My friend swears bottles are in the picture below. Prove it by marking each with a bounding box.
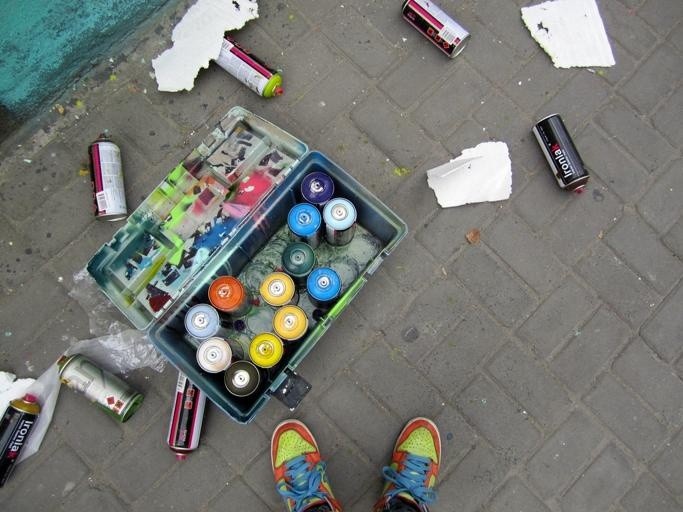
[57,354,146,424]
[89,132,129,223]
[280,242,319,289]
[306,267,343,310]
[322,196,357,247]
[299,171,335,207]
[165,371,208,461]
[286,202,322,252]
[531,112,590,196]
[0,393,41,489]
[401,0,472,59]
[182,271,309,401]
[212,34,283,100]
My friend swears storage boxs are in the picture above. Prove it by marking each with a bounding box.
[82,105,415,428]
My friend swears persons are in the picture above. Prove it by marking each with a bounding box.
[269,415,443,512]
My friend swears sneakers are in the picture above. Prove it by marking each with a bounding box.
[271,418,342,512]
[377,416,441,512]
